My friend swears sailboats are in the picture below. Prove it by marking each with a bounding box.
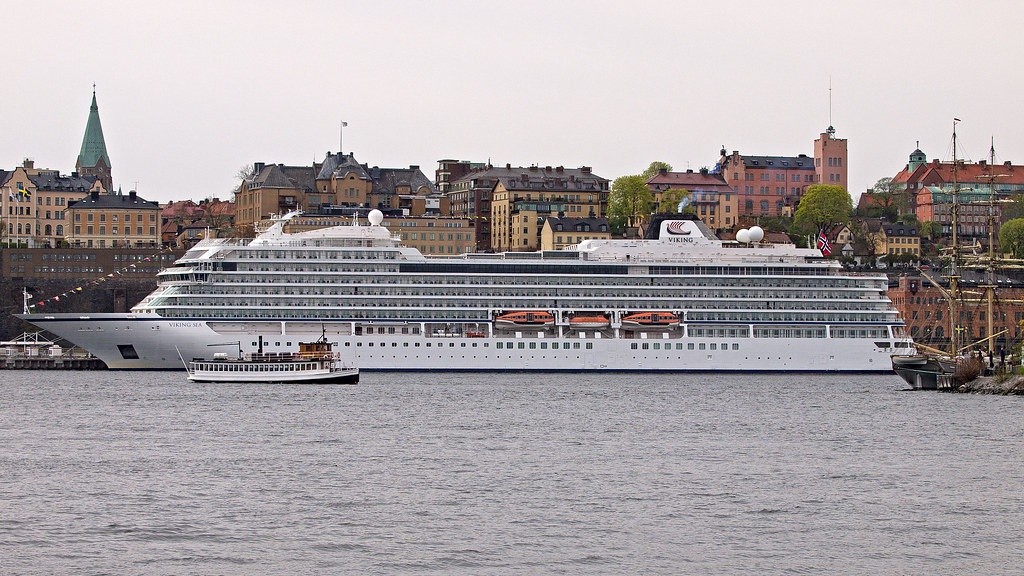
[971,134,1008,375]
[893,120,973,391]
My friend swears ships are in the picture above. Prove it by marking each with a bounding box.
[14,209,923,376]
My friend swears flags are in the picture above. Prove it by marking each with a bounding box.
[817,232,833,256]
[342,122,347,127]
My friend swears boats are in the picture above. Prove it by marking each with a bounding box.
[622,311,679,323]
[493,310,554,330]
[569,313,609,328]
[185,322,361,387]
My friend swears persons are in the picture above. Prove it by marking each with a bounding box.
[1000,348,1005,365]
[978,349,982,357]
[987,349,994,368]
